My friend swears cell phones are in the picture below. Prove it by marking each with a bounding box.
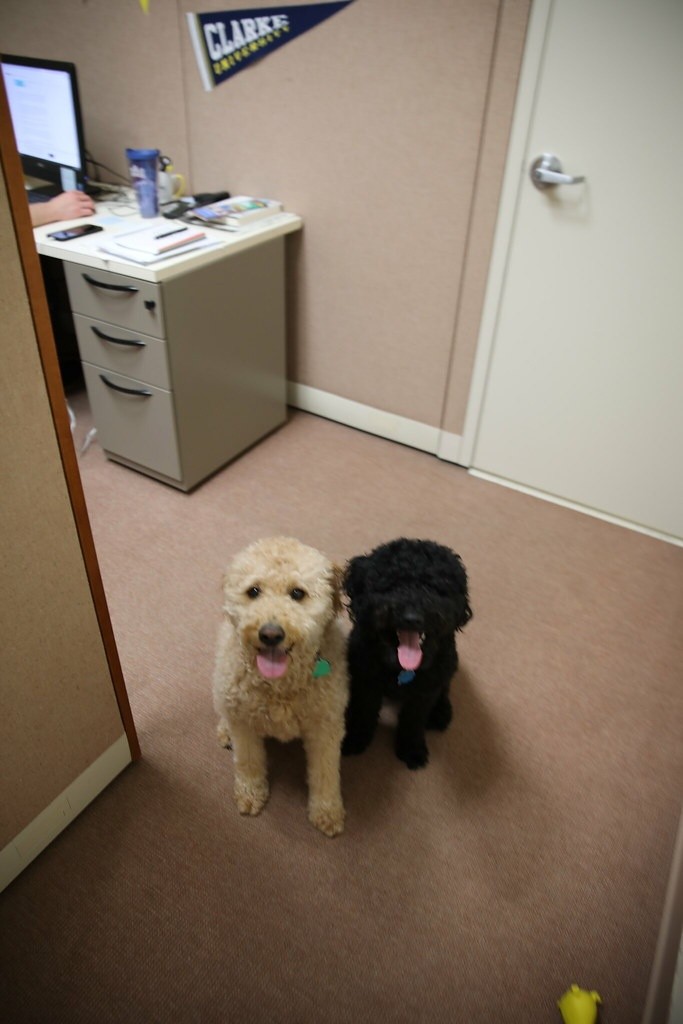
[48,224,102,241]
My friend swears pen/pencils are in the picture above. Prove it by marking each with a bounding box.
[156,227,187,239]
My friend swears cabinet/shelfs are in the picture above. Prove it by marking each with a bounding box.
[62,234,287,492]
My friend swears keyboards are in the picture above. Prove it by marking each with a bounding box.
[26,190,51,205]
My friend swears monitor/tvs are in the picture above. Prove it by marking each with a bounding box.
[0,53,101,200]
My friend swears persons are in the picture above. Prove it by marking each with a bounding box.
[30,189,99,228]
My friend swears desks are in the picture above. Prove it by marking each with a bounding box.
[20,161,304,285]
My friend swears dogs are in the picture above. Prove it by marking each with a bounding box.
[208,535,475,841]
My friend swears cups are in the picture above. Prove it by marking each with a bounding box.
[126,148,158,218]
[157,172,185,203]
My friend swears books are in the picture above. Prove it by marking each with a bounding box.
[96,195,285,265]
